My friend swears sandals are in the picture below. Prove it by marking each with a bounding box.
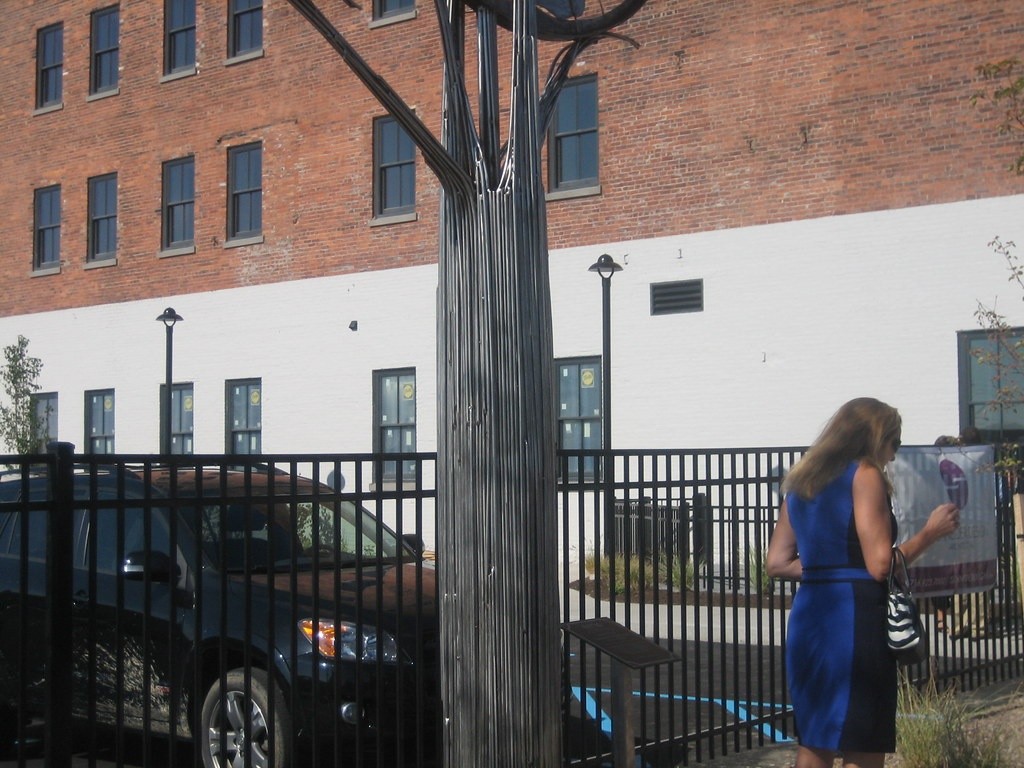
[938,620,949,632]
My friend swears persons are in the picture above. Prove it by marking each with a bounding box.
[923,425,1023,643]
[763,397,959,768]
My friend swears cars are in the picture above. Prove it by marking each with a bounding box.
[0,456,440,768]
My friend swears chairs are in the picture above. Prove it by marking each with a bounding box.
[224,504,269,568]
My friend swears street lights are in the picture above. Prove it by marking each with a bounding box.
[589,256,624,560]
[156,308,182,455]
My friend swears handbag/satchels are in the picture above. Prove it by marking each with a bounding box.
[888,548,929,665]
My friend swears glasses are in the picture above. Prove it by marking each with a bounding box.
[890,438,901,452]
